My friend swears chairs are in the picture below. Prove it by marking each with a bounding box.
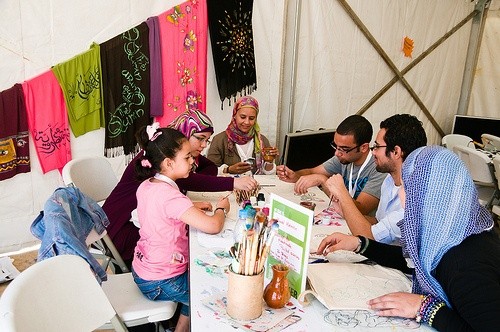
[60,155,129,272]
[0,254,129,332]
[441,133,500,217]
[44,185,178,332]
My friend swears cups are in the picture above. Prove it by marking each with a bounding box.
[226,263,265,321]
[261,146,276,162]
[300,201,316,211]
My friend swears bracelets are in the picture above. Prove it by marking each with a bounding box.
[416,293,445,327]
[226,166,229,173]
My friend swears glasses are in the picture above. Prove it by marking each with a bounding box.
[330,141,364,154]
[373,142,393,150]
[192,134,210,145]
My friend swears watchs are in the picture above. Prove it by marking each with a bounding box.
[214,207,227,218]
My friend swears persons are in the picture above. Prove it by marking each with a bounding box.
[317,144,500,332]
[99,96,279,331]
[277,115,429,245]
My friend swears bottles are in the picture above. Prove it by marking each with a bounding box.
[233,193,270,244]
[262,263,291,310]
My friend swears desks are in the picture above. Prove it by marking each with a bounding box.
[189,175,438,332]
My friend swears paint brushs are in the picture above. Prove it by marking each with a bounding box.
[228,199,279,275]
[225,191,233,199]
[283,159,287,172]
[327,193,334,211]
